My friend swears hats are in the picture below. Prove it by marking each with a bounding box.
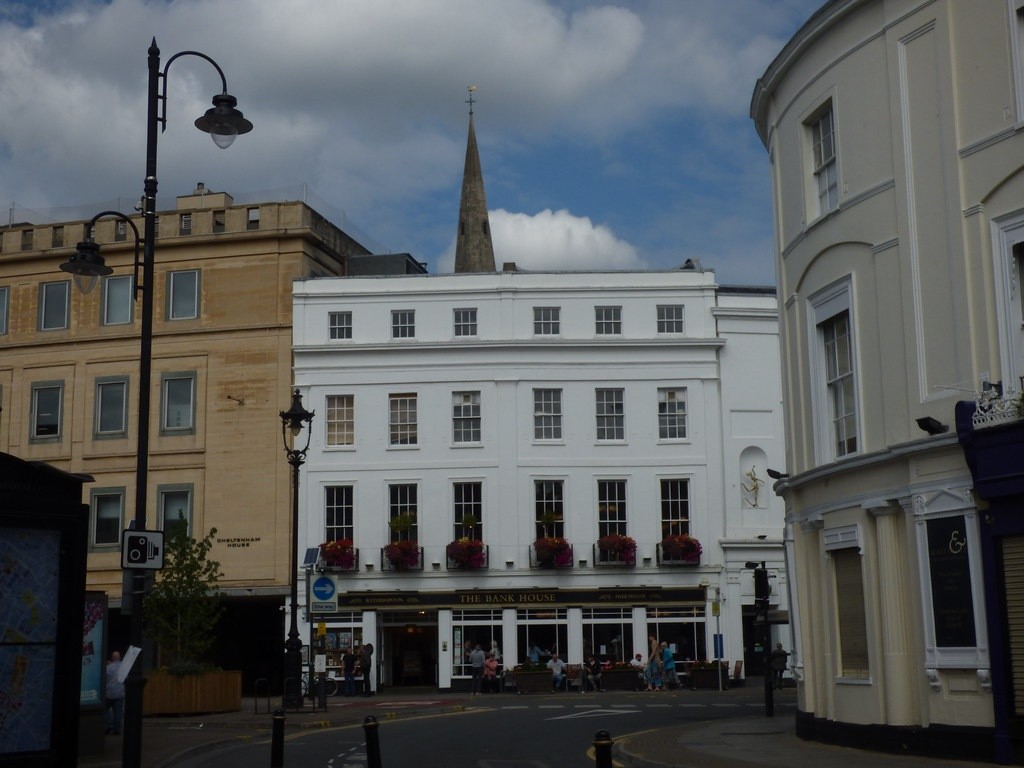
[636,654,643,658]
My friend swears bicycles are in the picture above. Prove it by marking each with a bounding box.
[300,671,337,698]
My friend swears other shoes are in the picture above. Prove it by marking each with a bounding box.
[470,692,482,696]
[632,689,670,692]
[592,688,607,693]
[678,684,683,691]
[106,728,120,736]
[555,689,559,692]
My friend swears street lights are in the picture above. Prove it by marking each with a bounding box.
[301,546,321,699]
[61,52,253,767]
[280,388,314,711]
[745,562,776,718]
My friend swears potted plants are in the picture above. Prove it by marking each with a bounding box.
[143,507,242,717]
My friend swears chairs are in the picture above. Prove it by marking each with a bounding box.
[543,663,603,693]
[479,664,525,694]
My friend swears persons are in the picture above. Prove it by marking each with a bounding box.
[105,652,124,736]
[528,642,548,662]
[583,654,607,693]
[772,642,787,690]
[358,645,371,694]
[341,647,358,697]
[628,633,685,692]
[464,640,501,695]
[546,654,566,692]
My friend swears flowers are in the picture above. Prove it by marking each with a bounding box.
[532,538,573,568]
[600,660,637,671]
[318,537,354,569]
[597,534,638,565]
[446,536,487,572]
[662,533,703,563]
[684,659,730,669]
[384,540,420,566]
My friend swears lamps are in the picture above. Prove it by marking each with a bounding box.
[766,469,789,479]
[915,417,949,435]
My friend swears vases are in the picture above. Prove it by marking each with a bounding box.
[602,671,641,690]
[691,668,728,690]
